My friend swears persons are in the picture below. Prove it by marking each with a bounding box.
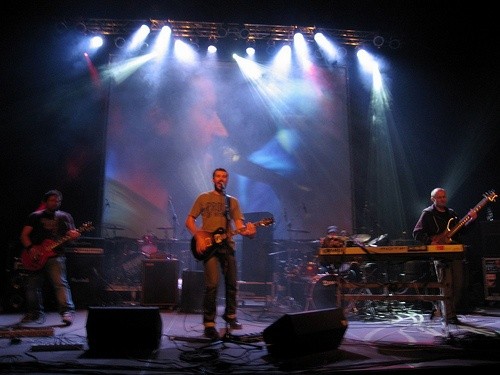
[185,168,256,338]
[22,191,81,324]
[327,226,348,236]
[413,189,488,312]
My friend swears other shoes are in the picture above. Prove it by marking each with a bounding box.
[61,313,72,324]
[221,313,242,330]
[21,314,36,322]
[204,325,216,333]
[446,317,461,326]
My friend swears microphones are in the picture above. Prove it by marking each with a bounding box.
[106,199,111,208]
[220,182,225,189]
[301,202,307,214]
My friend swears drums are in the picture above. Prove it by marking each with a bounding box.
[309,268,340,308]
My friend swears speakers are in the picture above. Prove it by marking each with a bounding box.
[263,308,347,359]
[86,308,163,353]
[140,260,206,310]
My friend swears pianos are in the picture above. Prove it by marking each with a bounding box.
[313,243,467,324]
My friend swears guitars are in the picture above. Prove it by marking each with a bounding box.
[21,220,95,271]
[190,215,276,260]
[428,191,497,256]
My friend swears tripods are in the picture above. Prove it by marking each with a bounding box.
[195,187,262,351]
